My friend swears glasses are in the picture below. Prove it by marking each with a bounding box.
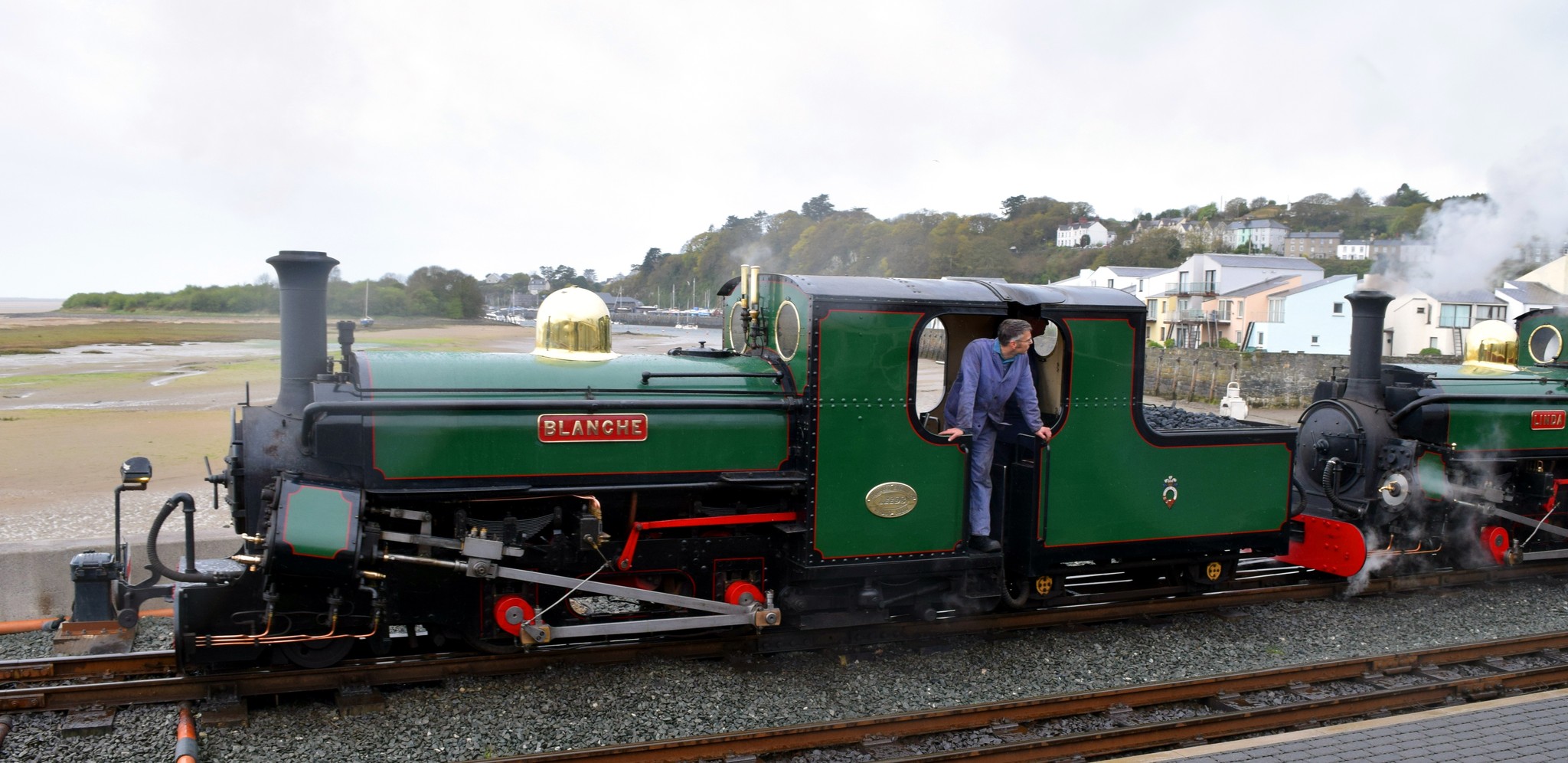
[1015,339,1032,344]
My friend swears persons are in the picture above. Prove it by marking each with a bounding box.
[938,319,1052,553]
[1004,317,1049,449]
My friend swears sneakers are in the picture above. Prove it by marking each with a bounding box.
[968,535,1001,552]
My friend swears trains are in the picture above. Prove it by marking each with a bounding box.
[1270,285,1568,581]
[116,246,1297,629]
